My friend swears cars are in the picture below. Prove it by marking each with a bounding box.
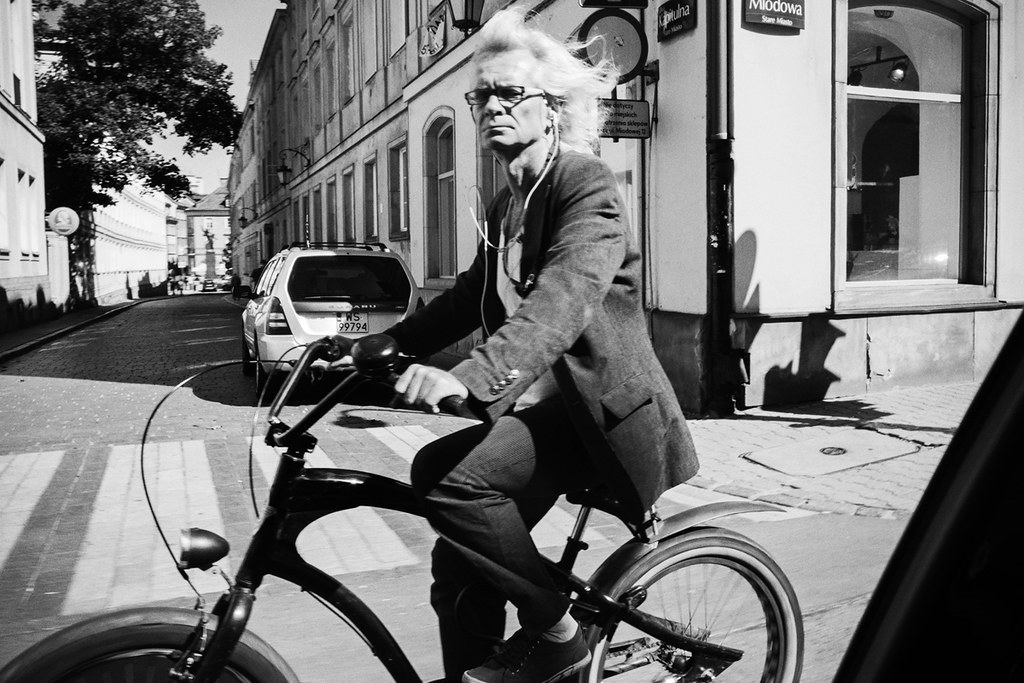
[169,267,233,292]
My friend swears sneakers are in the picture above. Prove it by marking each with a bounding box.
[461,620,593,683]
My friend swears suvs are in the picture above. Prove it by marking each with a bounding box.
[233,241,426,399]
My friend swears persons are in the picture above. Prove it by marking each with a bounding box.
[331,8,700,683]
[170,278,185,294]
[203,230,213,249]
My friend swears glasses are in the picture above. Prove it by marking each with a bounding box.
[464,85,548,106]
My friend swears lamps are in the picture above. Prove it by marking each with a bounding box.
[887,58,909,83]
[276,149,313,187]
[848,68,863,85]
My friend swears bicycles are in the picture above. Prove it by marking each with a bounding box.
[0,333,809,683]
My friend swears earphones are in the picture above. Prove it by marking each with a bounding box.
[550,109,558,126]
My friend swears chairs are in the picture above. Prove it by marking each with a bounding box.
[342,277,379,300]
[291,276,326,301]
